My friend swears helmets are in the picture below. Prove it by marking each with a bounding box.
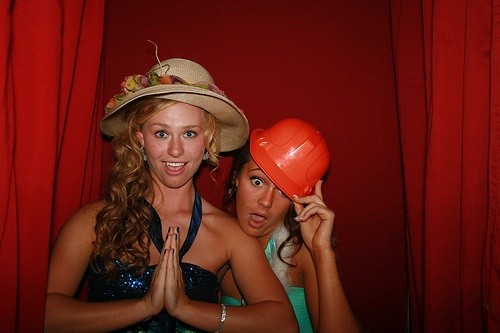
[250,118,331,201]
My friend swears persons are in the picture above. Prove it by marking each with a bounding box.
[44,57,300,333]
[217,118,362,333]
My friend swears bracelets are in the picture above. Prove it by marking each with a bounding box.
[214,304,226,333]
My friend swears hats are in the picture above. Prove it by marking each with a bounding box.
[99,58,249,153]
